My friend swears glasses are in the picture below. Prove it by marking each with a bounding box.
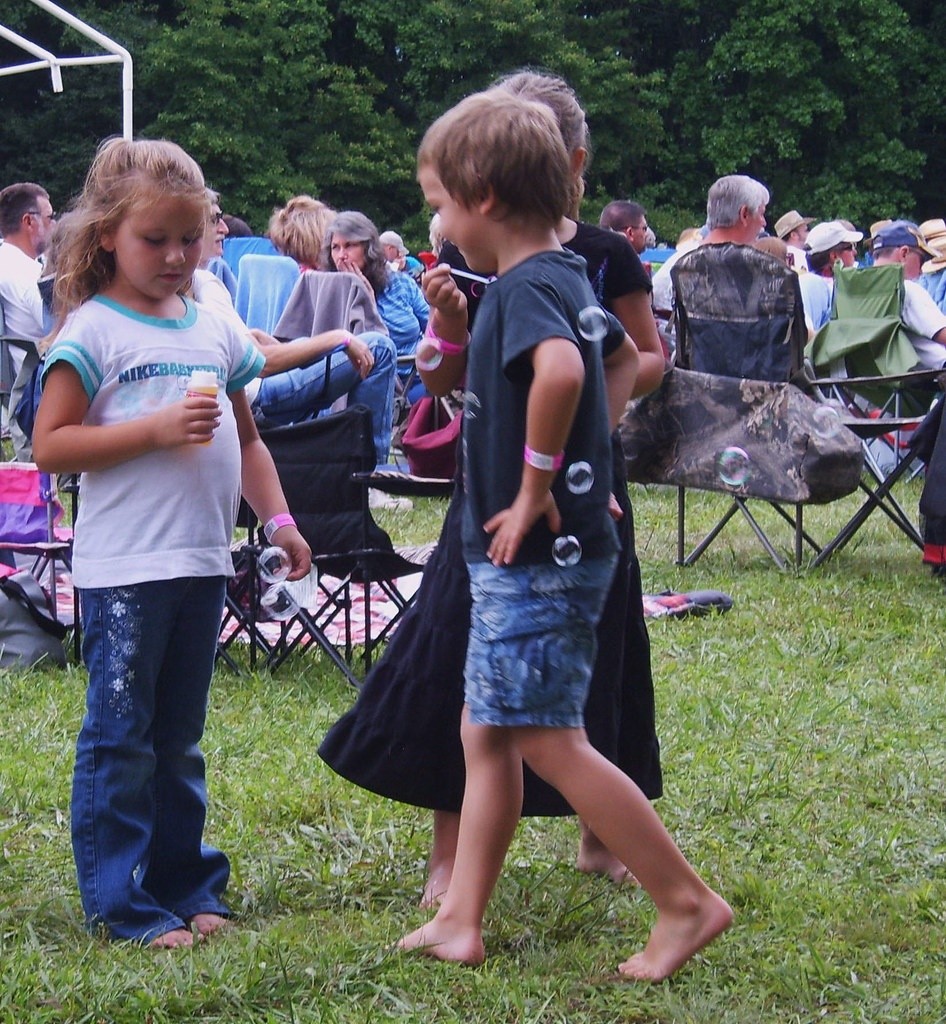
[20,209,58,224]
[896,246,926,267]
[619,224,649,236]
[208,210,225,225]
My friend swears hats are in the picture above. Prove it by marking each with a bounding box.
[873,220,942,259]
[775,211,816,240]
[379,230,409,256]
[921,233,946,271]
[803,222,864,256]
[864,220,893,246]
[918,219,946,240]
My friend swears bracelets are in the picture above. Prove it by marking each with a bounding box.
[264,514,297,542]
[523,445,564,472]
[425,323,471,356]
[343,330,351,346]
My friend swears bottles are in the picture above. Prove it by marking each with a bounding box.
[186,370,218,446]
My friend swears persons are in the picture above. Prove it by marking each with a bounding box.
[651,174,814,342]
[0,183,58,375]
[599,201,649,254]
[316,72,664,914]
[34,140,312,948]
[754,210,946,346]
[267,196,430,403]
[190,186,397,466]
[387,91,734,984]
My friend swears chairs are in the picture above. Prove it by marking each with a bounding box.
[0,238,945,689]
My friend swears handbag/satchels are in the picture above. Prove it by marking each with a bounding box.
[0,570,71,677]
